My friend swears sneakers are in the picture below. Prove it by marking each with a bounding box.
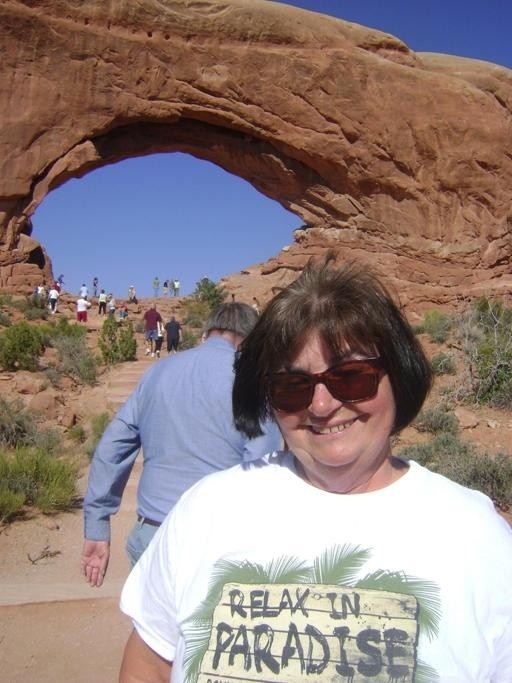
[144,348,162,359]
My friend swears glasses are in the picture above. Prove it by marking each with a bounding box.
[261,353,390,414]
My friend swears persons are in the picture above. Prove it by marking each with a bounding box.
[79,300,285,594]
[37,271,264,357]
[114,253,511,683]
